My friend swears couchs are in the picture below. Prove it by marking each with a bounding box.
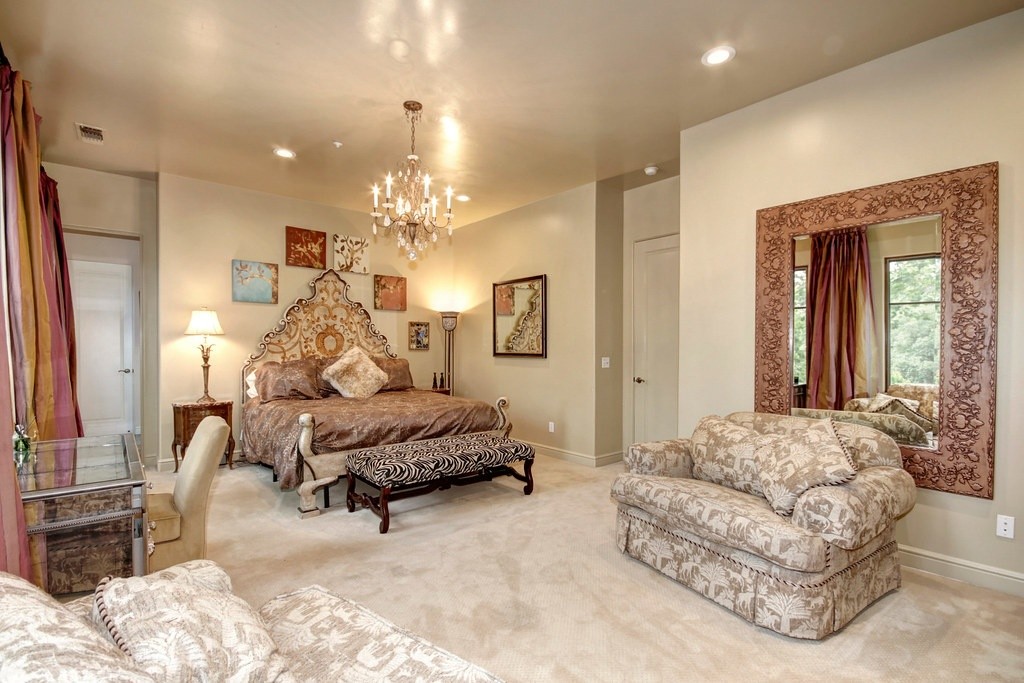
[792,407,928,446]
[610,411,919,640]
[0,558,507,681]
[844,381,938,418]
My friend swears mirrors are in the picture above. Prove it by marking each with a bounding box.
[750,161,1003,498]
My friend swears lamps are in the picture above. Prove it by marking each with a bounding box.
[184,308,224,402]
[367,100,471,260]
[440,311,460,396]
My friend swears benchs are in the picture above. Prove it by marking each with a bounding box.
[346,428,535,534]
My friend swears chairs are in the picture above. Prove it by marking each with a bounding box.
[137,415,229,574]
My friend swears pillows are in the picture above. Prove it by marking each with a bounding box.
[872,399,933,432]
[367,357,413,391]
[868,391,920,413]
[754,419,851,516]
[91,557,294,681]
[249,355,318,401]
[316,353,349,396]
[689,414,789,492]
[321,346,389,397]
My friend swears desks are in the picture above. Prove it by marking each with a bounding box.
[19,432,151,593]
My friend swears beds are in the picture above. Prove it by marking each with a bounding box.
[242,267,508,508]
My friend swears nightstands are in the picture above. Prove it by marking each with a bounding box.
[422,384,452,394]
[168,400,235,471]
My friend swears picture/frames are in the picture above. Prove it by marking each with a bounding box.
[493,274,546,355]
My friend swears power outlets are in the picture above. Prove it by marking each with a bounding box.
[996,515,1015,539]
[548,422,554,432]
